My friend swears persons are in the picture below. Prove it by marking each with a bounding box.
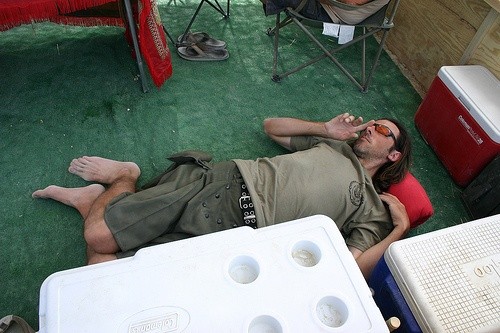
[29,109,413,283]
[259,0,391,29]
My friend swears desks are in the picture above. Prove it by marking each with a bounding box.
[0,0,172,93]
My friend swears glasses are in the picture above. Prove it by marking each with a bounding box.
[372,123,397,146]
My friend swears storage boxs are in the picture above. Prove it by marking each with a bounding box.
[367,213,500,333]
[39,213,391,333]
[413,64,500,190]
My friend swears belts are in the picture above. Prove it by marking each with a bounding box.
[238,180,258,231]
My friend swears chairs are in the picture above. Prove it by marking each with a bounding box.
[265,0,400,94]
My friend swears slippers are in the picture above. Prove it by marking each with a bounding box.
[177,46,230,61]
[177,31,227,48]
